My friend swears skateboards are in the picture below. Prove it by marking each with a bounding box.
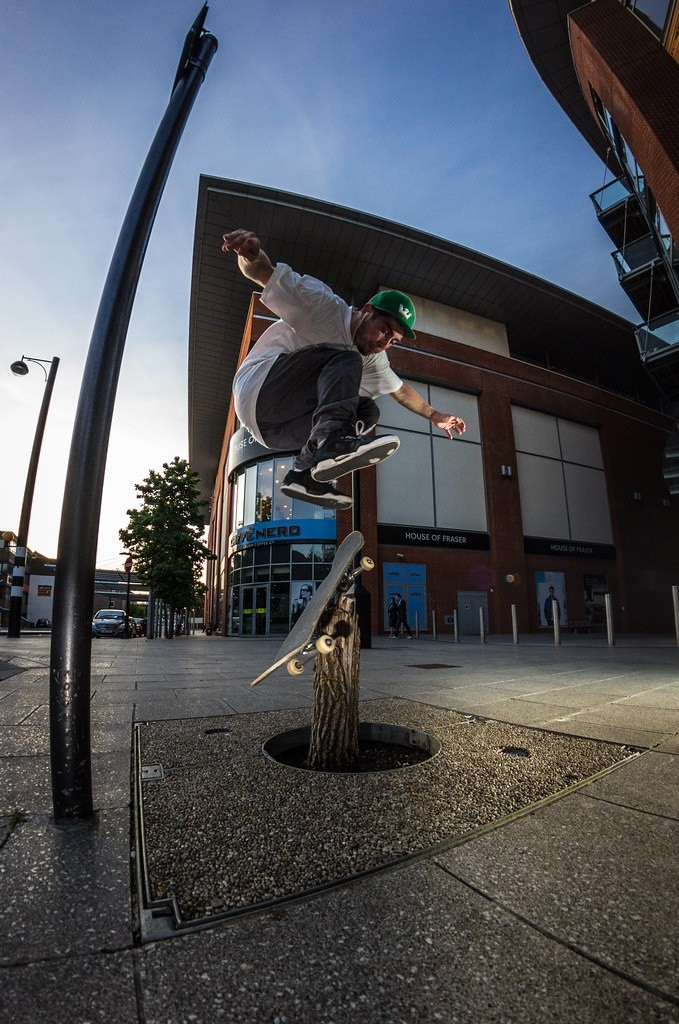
[251,530,377,685]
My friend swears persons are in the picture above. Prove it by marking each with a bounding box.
[222,228,466,510]
[544,586,561,626]
[391,594,413,639]
[291,584,313,630]
[386,597,398,639]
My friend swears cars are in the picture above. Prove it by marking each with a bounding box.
[128,617,135,637]
[135,618,147,636]
[36,619,50,628]
[92,609,126,638]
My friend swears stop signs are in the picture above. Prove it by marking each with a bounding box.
[125,556,133,571]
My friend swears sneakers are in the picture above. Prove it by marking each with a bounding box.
[309,421,400,483]
[280,466,353,511]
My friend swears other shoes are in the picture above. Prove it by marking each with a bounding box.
[406,636,414,640]
[389,635,398,638]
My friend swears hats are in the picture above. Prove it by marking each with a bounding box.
[367,290,417,340]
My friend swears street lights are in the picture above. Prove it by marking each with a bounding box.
[8,355,61,637]
